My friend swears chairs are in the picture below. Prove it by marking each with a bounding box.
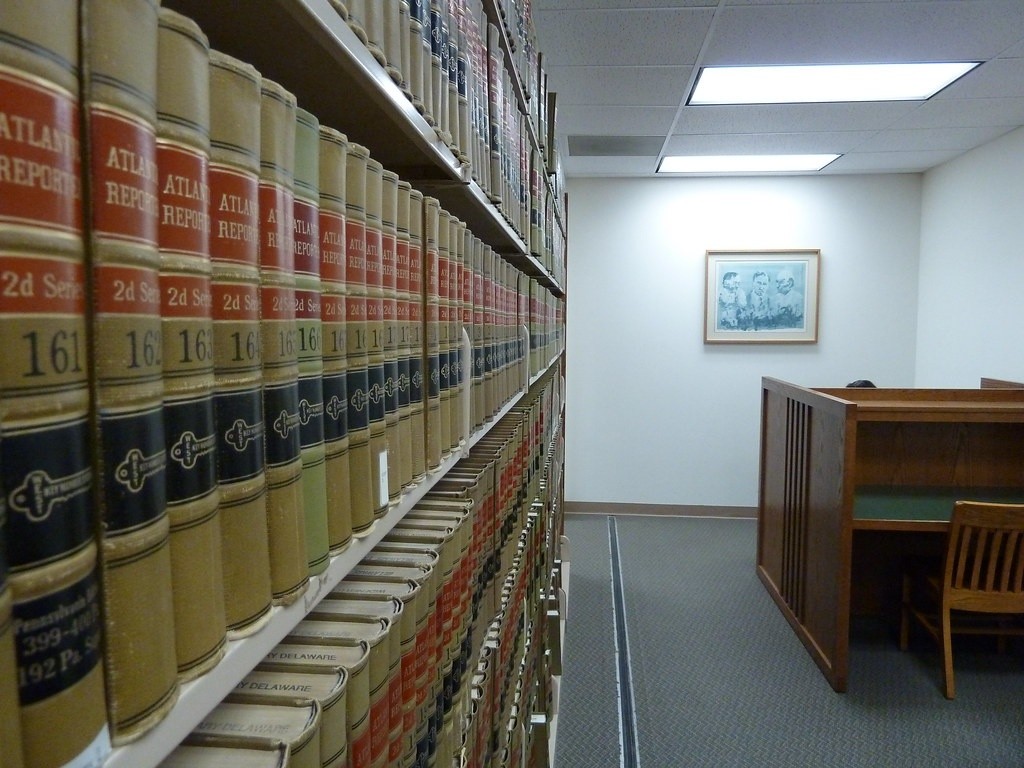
[898,501,1024,700]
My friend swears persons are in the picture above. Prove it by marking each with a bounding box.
[720,271,802,313]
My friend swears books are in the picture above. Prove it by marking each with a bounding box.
[1,0,349,767]
[161,356,566,768]
[330,0,568,559]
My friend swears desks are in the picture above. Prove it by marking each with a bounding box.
[852,485,1024,532]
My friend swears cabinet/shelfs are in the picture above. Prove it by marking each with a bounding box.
[0,0,570,768]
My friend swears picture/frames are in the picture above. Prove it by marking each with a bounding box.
[703,248,821,343]
